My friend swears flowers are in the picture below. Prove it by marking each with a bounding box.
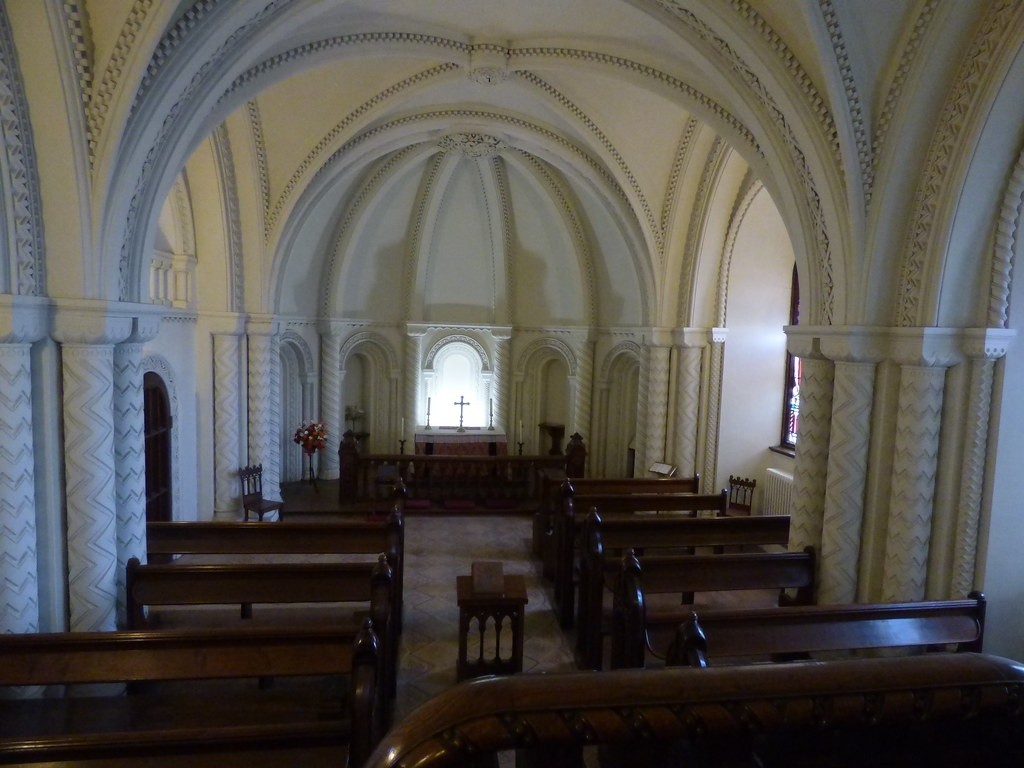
[295,420,333,455]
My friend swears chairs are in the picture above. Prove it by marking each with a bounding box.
[718,475,757,517]
[238,464,284,522]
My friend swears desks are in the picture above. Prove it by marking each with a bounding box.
[457,574,528,682]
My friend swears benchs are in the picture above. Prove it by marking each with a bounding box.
[0,515,405,768]
[532,473,987,674]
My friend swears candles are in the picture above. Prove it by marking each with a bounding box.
[401,417,404,441]
[427,397,431,415]
[519,419,522,444]
[490,398,492,415]
